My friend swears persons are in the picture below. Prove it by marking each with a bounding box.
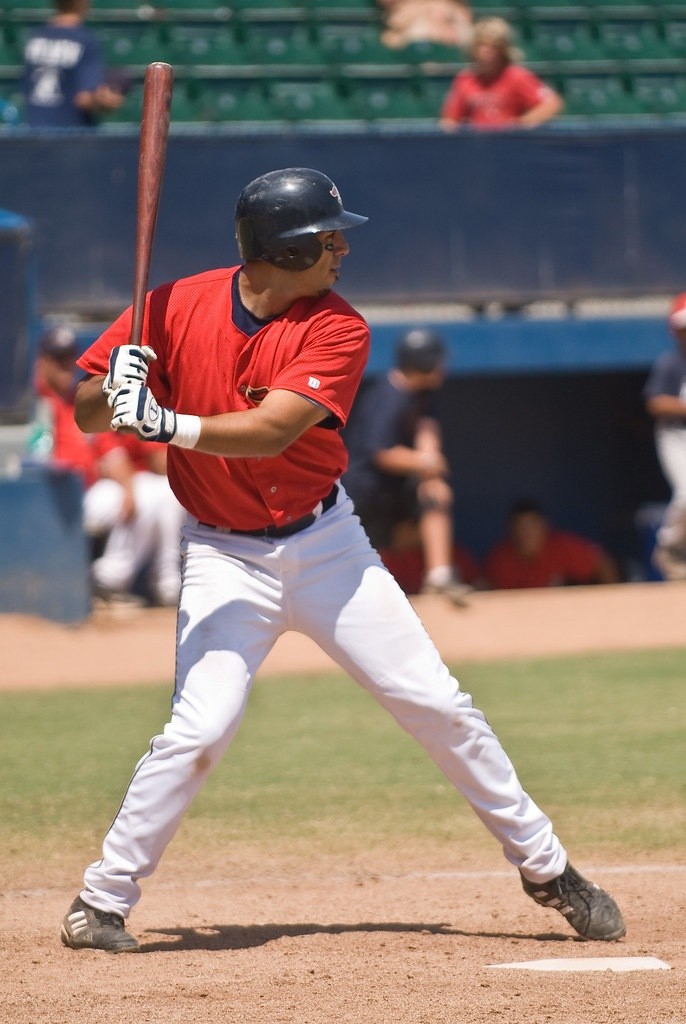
[60,166,630,955]
[436,16,564,134]
[19,0,134,127]
[380,0,524,97]
[31,283,685,609]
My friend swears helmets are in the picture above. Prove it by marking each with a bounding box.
[392,329,443,370]
[235,168,370,272]
[42,328,81,360]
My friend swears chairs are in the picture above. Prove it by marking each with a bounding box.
[0,0,686,126]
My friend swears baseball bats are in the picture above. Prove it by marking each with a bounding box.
[114,62,178,436]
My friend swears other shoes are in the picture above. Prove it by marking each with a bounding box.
[421,578,472,609]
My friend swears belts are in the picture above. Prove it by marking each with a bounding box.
[199,483,340,539]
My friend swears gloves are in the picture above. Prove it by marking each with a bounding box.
[107,383,202,450]
[102,344,158,396]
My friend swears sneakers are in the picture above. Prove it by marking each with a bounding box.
[61,895,140,952]
[518,859,626,941]
